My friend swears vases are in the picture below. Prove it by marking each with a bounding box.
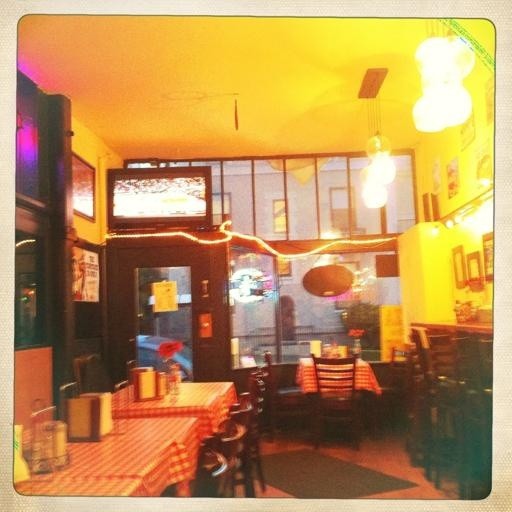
[353,339,361,354]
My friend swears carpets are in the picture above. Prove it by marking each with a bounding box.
[249,447,418,499]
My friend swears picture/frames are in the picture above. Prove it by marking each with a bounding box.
[483,232,494,283]
[452,247,466,289]
[466,251,482,283]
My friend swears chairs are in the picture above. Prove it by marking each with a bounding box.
[309,350,365,449]
[245,375,267,492]
[264,351,304,442]
[455,331,493,501]
[13,415,196,498]
[72,150,96,224]
[412,328,464,489]
[199,447,231,497]
[76,352,107,391]
[228,393,256,499]
[58,381,84,417]
[427,334,450,434]
[220,423,248,499]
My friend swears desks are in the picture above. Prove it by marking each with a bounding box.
[95,382,236,442]
[300,353,370,394]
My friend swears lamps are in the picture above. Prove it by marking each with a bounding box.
[358,152,396,211]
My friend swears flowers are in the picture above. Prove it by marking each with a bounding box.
[347,328,364,339]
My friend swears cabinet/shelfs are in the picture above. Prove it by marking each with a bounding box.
[17,68,48,203]
